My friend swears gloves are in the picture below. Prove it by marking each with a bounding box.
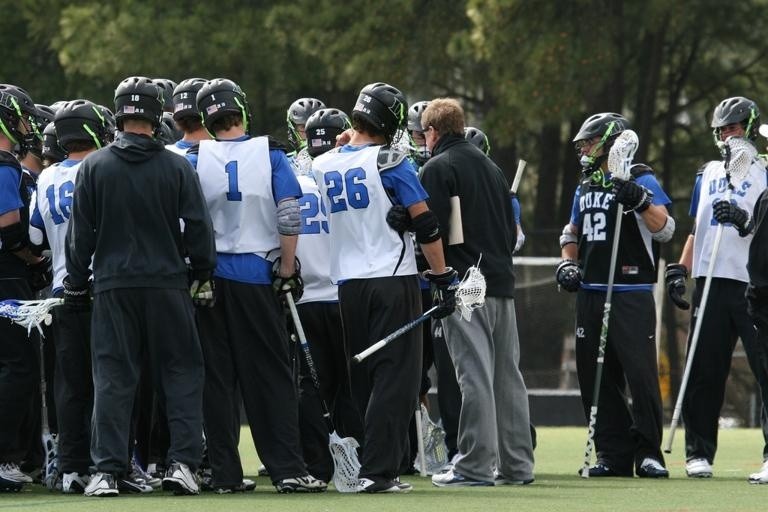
[271,256,304,307]
[424,267,459,320]
[664,263,690,310]
[62,275,92,331]
[712,199,755,237]
[611,176,654,214]
[554,260,585,293]
[189,280,217,309]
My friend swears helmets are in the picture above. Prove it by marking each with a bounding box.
[286,97,352,156]
[51,100,68,114]
[114,77,249,153]
[352,82,490,156]
[571,112,632,179]
[0,84,114,161]
[711,96,760,160]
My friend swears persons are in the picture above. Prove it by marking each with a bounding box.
[665,96,768,484]
[0,77,536,496]
[555,112,675,478]
[713,123,768,482]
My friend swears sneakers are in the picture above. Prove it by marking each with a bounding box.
[116,477,153,494]
[578,464,633,477]
[162,461,200,495]
[431,469,496,487]
[129,464,162,488]
[357,478,413,493]
[685,457,713,478]
[214,479,256,494]
[749,459,768,484]
[63,471,86,494]
[41,434,70,490]
[635,458,669,478]
[201,469,213,492]
[0,462,34,483]
[276,474,328,494]
[258,465,270,477]
[494,475,535,485]
[83,471,120,497]
